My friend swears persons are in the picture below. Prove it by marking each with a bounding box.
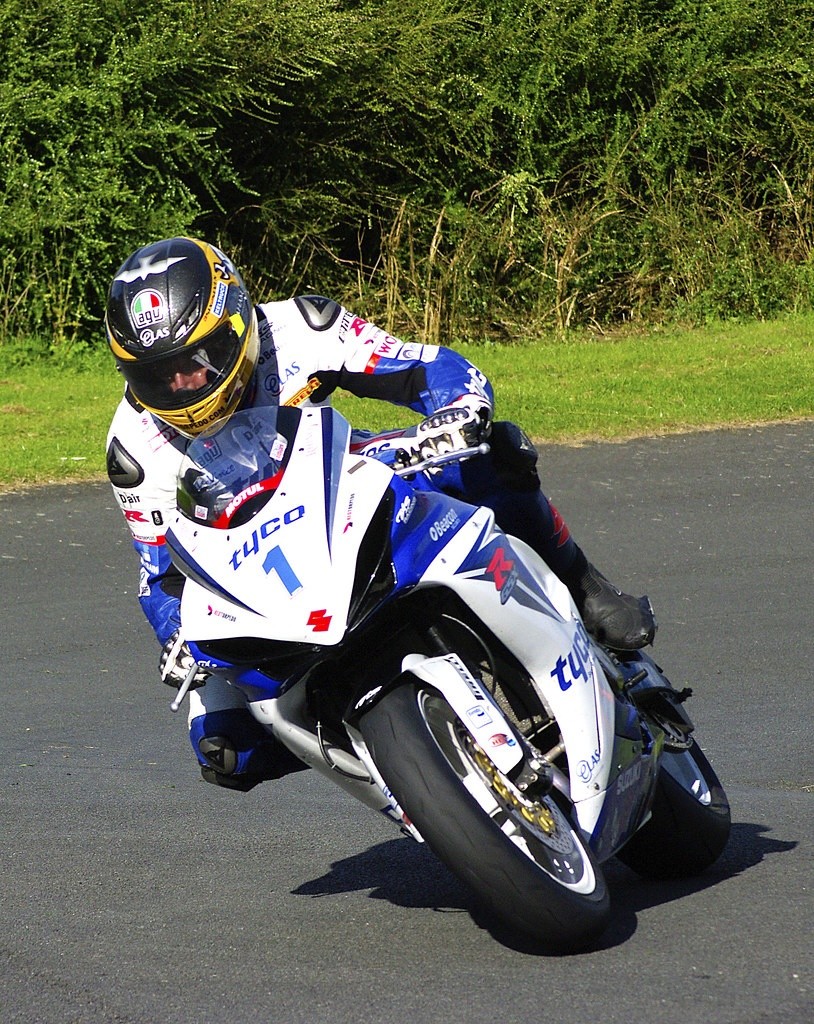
[105,235,655,794]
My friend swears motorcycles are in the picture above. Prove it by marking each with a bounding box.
[160,405,732,951]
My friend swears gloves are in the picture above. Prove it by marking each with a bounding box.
[158,631,214,692]
[417,393,494,477]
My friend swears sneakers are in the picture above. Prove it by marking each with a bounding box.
[557,541,655,651]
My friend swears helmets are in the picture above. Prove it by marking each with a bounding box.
[105,238,260,440]
